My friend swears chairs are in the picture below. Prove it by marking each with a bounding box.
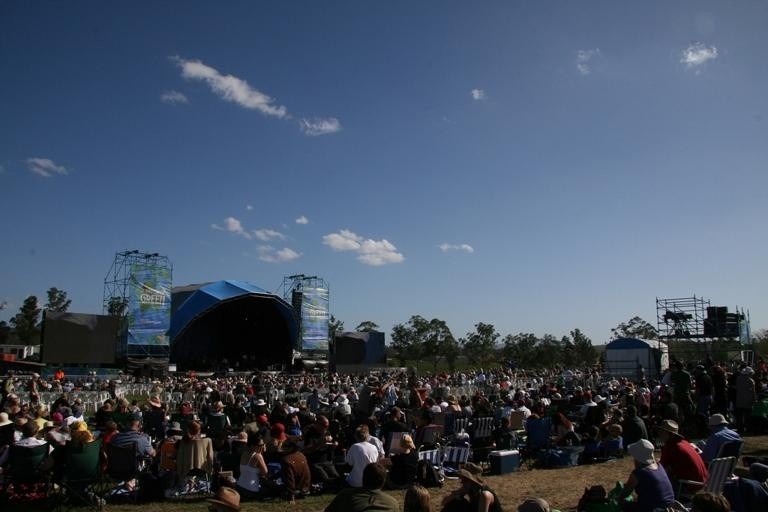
[674,456,739,511]
[104,439,142,498]
[513,413,552,469]
[154,403,252,503]
[0,420,114,512]
[382,413,498,490]
[700,437,743,467]
[552,435,625,471]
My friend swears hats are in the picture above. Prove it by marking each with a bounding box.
[456,463,485,487]
[337,394,349,405]
[741,366,756,375]
[146,396,163,408]
[71,422,87,432]
[429,394,458,404]
[626,439,657,464]
[550,387,608,408]
[43,413,63,429]
[214,397,284,436]
[653,418,687,440]
[117,397,130,407]
[14,418,27,425]
[206,485,241,510]
[168,421,183,432]
[706,414,730,427]
[32,372,41,378]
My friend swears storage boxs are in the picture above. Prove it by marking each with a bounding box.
[489,447,520,473]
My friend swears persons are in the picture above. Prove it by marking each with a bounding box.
[0,352,766,512]
